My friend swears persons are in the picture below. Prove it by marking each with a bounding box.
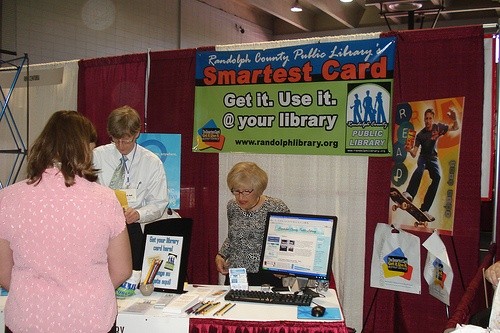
[215,163,290,286]
[91,106,169,272]
[0,109,132,333]
[448,261,500,333]
[402,109,459,221]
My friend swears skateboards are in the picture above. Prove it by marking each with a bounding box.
[390,187,432,228]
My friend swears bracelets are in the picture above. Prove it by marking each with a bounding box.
[135,211,141,220]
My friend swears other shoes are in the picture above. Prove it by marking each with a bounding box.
[422,211,436,221]
[403,191,413,202]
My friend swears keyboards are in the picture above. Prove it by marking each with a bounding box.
[225,290,313,306]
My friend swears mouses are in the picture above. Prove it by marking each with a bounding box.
[312,306,325,317]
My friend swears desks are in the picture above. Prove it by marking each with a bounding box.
[0,284,347,333]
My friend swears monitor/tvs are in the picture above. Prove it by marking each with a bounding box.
[259,212,338,297]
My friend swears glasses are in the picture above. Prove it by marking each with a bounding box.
[230,187,254,195]
[110,134,137,145]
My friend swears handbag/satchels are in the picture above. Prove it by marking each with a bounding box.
[422,228,453,305]
[369,223,421,294]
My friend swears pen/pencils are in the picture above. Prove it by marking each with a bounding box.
[143,259,163,284]
[185,300,237,316]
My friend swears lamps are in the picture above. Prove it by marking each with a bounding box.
[290,0,304,13]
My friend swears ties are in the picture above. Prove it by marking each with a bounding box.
[109,155,128,189]
